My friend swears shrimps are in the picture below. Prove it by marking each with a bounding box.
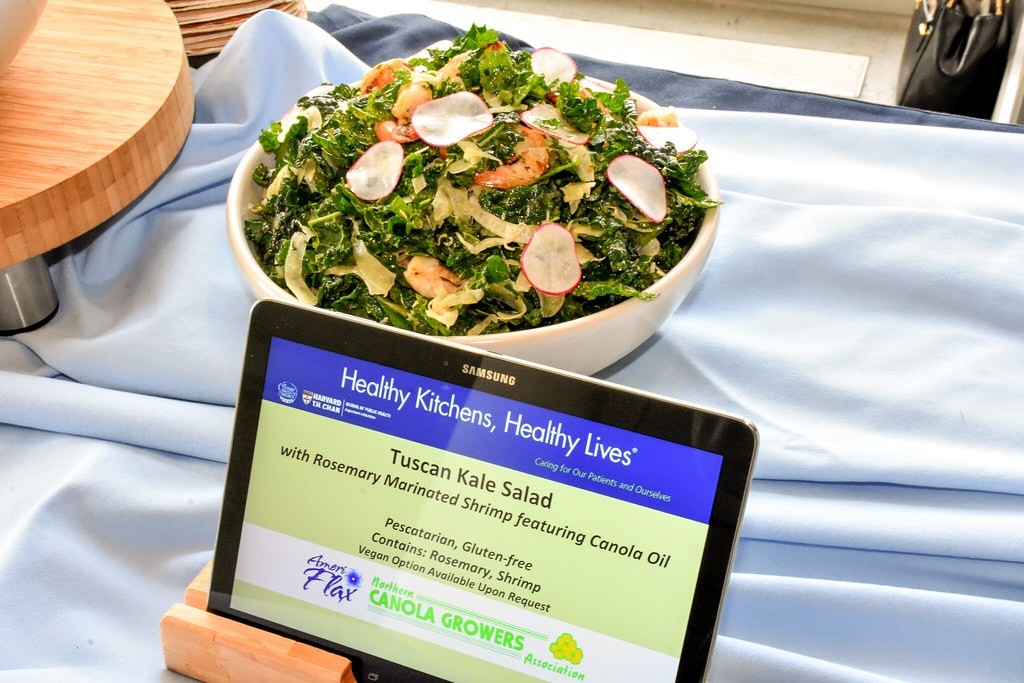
[639,105,679,127]
[393,87,433,125]
[483,40,503,52]
[473,120,549,189]
[372,118,419,143]
[438,146,449,159]
[403,254,463,298]
[362,58,414,95]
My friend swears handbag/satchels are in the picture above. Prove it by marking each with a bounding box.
[894,0,1020,121]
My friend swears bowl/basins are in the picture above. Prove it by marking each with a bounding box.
[229,73,720,375]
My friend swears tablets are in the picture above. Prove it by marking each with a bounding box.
[208,298,757,683]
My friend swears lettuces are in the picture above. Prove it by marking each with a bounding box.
[240,24,725,340]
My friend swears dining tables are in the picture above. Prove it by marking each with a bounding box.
[1,0,1023,681]
